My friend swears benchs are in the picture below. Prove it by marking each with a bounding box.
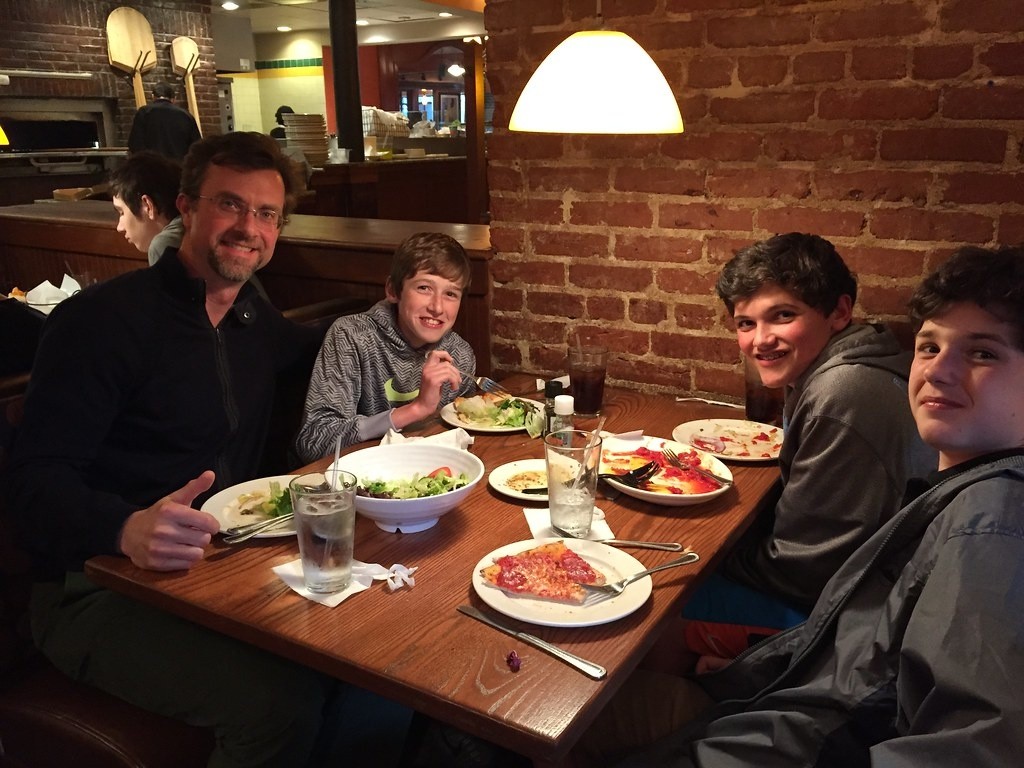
[0,296,369,768]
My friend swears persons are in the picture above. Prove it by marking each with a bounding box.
[536,244,1024,768]
[296,232,476,464]
[0,130,416,768]
[0,294,50,378]
[647,233,940,678]
[107,151,188,263]
[127,82,201,162]
[270,106,294,137]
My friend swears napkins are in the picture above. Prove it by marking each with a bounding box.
[378,427,474,452]
[580,429,645,439]
[270,556,394,608]
[522,505,615,543]
[536,374,571,391]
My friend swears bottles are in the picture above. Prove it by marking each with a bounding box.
[543,381,562,438]
[551,395,575,458]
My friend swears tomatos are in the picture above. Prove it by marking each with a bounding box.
[428,467,451,478]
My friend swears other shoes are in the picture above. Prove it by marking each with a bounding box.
[430,721,494,768]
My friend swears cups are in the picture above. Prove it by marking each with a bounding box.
[567,345,607,418]
[542,430,602,537]
[289,469,357,595]
[449,127,457,139]
[405,148,426,159]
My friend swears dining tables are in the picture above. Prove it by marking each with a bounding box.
[85,373,783,768]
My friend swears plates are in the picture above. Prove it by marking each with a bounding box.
[487,459,583,502]
[472,538,652,625]
[585,436,733,505]
[441,396,544,432]
[281,113,330,167]
[672,418,783,462]
[199,474,301,538]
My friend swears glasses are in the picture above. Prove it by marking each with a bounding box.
[186,191,289,230]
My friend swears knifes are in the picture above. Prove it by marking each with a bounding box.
[457,606,608,680]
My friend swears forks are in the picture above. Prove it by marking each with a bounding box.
[523,461,659,497]
[425,351,509,399]
[575,552,700,594]
[550,524,682,553]
[662,448,733,486]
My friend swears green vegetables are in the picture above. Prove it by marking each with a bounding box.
[337,470,470,500]
[457,395,545,440]
[236,480,308,520]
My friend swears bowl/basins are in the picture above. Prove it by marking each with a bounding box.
[326,148,351,165]
[324,443,485,535]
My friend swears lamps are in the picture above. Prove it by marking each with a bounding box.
[507,13,685,134]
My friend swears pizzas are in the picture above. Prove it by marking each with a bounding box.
[480,540,606,603]
